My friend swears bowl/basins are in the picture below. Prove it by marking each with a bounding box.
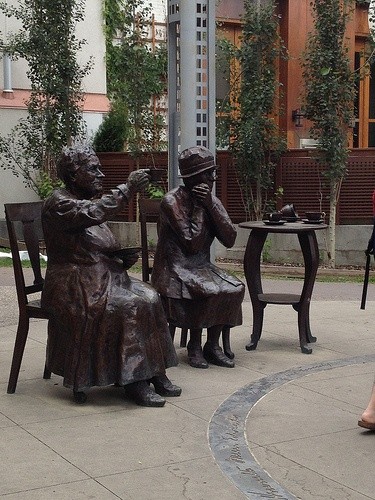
[279,204,296,216]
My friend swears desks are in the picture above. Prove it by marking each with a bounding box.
[239,220,328,353]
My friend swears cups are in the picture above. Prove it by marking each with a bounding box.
[306,212,326,220]
[266,213,283,222]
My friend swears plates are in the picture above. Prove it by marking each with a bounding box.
[303,220,326,223]
[112,247,142,254]
[263,220,287,225]
[280,217,300,221]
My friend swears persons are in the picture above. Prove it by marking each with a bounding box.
[42,144,183,407]
[150,146,246,369]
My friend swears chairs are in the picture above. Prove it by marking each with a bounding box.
[4,201,51,394]
[138,198,188,348]
